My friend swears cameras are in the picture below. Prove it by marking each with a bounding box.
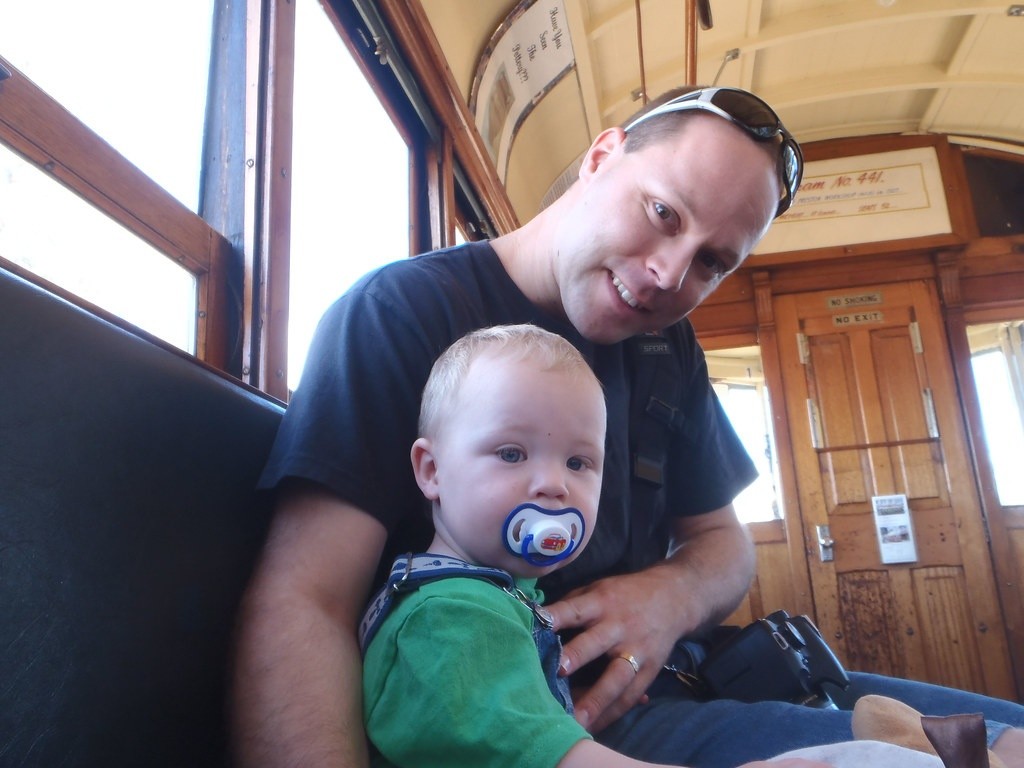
[694,609,851,711]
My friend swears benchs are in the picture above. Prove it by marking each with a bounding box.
[0,270,287,768]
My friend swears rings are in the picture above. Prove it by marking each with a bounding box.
[620,654,638,672]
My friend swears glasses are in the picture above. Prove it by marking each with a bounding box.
[623,88,805,220]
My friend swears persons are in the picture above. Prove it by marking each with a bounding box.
[362,325,833,768]
[231,87,1024,768]
